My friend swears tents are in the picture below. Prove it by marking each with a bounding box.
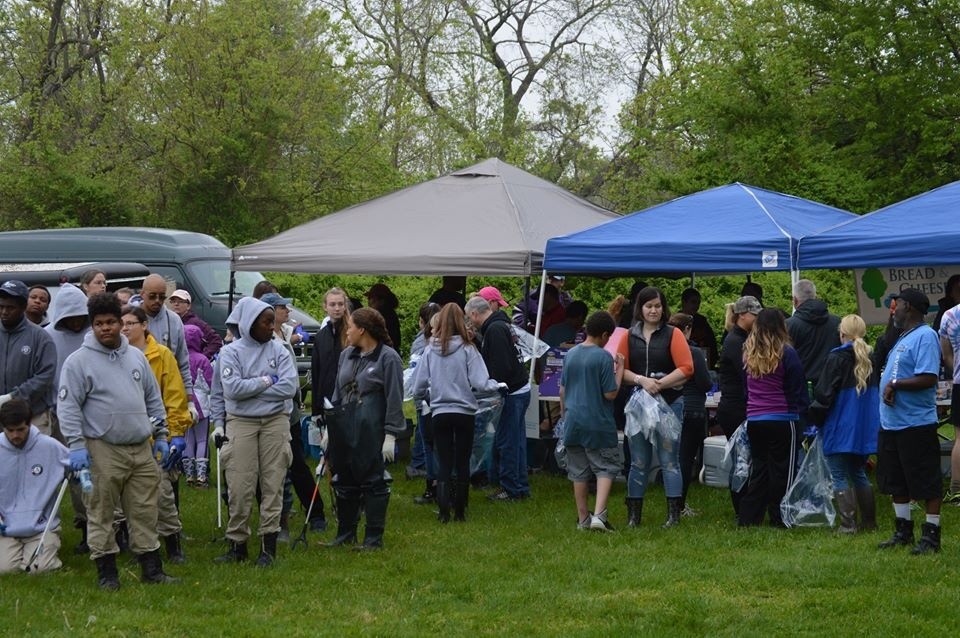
[528,180,860,390]
[231,156,623,284]
[797,180,960,273]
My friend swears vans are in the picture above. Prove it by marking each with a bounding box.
[0,228,324,407]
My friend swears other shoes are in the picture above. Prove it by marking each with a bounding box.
[576,507,616,533]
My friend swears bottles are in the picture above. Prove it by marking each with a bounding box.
[807,381,812,406]
[80,466,93,494]
[650,373,684,390]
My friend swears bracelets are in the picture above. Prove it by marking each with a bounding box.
[634,375,643,385]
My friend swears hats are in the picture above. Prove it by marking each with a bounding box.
[733,296,765,314]
[527,272,566,299]
[888,287,930,317]
[0,280,31,303]
[260,293,294,306]
[362,281,390,298]
[680,288,699,314]
[167,290,193,303]
[477,286,511,309]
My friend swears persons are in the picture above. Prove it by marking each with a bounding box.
[742,308,811,528]
[315,308,404,552]
[212,299,298,568]
[559,312,625,532]
[813,314,881,534]
[25,269,221,556]
[616,287,694,529]
[57,295,177,591]
[119,307,193,564]
[0,280,58,438]
[878,288,943,557]
[0,400,79,577]
[223,274,960,543]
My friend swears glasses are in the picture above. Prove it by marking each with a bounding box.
[142,290,191,306]
[87,280,108,286]
[120,321,145,327]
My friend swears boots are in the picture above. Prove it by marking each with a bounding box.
[70,521,185,593]
[214,464,530,569]
[182,457,211,489]
[834,485,960,556]
[620,495,684,530]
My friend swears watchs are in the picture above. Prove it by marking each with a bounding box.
[891,379,897,389]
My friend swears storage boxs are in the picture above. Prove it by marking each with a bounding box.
[699,435,729,487]
[617,431,624,469]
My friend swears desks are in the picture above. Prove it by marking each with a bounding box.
[936,399,952,441]
[705,392,722,439]
[539,395,561,445]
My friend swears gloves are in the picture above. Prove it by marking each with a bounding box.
[496,382,511,390]
[148,401,226,473]
[69,447,92,471]
[309,411,331,456]
[381,435,396,463]
